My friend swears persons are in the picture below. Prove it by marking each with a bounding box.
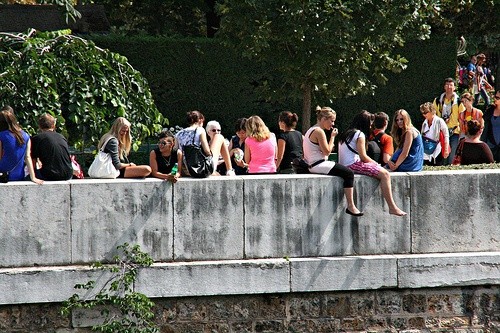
[173,112,280,178]
[433,53,500,164]
[69,156,84,179]
[0,110,44,186]
[26,113,73,181]
[455,120,495,165]
[276,111,304,172]
[1,105,14,113]
[369,112,394,164]
[418,103,450,166]
[338,110,407,216]
[387,109,424,171]
[98,117,152,178]
[150,130,182,183]
[302,105,364,217]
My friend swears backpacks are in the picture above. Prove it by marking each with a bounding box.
[368,132,385,167]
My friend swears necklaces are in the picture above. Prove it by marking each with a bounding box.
[164,157,172,167]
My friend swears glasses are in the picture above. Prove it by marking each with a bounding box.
[395,118,403,122]
[494,96,500,100]
[421,111,429,115]
[158,140,171,145]
[462,99,469,103]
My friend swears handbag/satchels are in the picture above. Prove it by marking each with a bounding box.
[88,137,120,180]
[0,171,11,183]
[452,154,462,165]
[70,155,85,179]
[183,143,214,178]
[292,158,312,175]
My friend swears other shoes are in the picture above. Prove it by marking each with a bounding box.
[345,208,364,217]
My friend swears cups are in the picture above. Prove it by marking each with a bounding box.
[234,148,244,163]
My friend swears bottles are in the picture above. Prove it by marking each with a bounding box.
[24,165,30,177]
[171,163,178,177]
